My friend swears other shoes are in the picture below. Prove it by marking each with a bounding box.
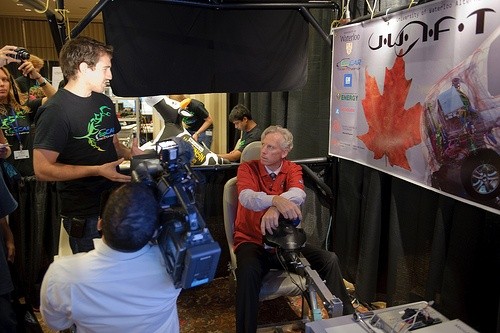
[31,303,39,311]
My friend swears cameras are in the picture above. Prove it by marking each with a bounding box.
[10,47,30,62]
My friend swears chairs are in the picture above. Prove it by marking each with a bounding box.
[222,141,334,321]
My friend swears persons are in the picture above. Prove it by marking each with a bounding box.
[216,104,264,161]
[168,94,214,185]
[0,45,58,333]
[233,126,355,333]
[32,35,146,255]
[40,183,183,333]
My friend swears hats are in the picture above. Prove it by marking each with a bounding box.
[102,182,157,251]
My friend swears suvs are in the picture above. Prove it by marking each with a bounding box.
[420,21,500,203]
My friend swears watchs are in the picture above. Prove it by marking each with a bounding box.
[38,78,47,86]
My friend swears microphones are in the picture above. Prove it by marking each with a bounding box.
[173,137,194,177]
